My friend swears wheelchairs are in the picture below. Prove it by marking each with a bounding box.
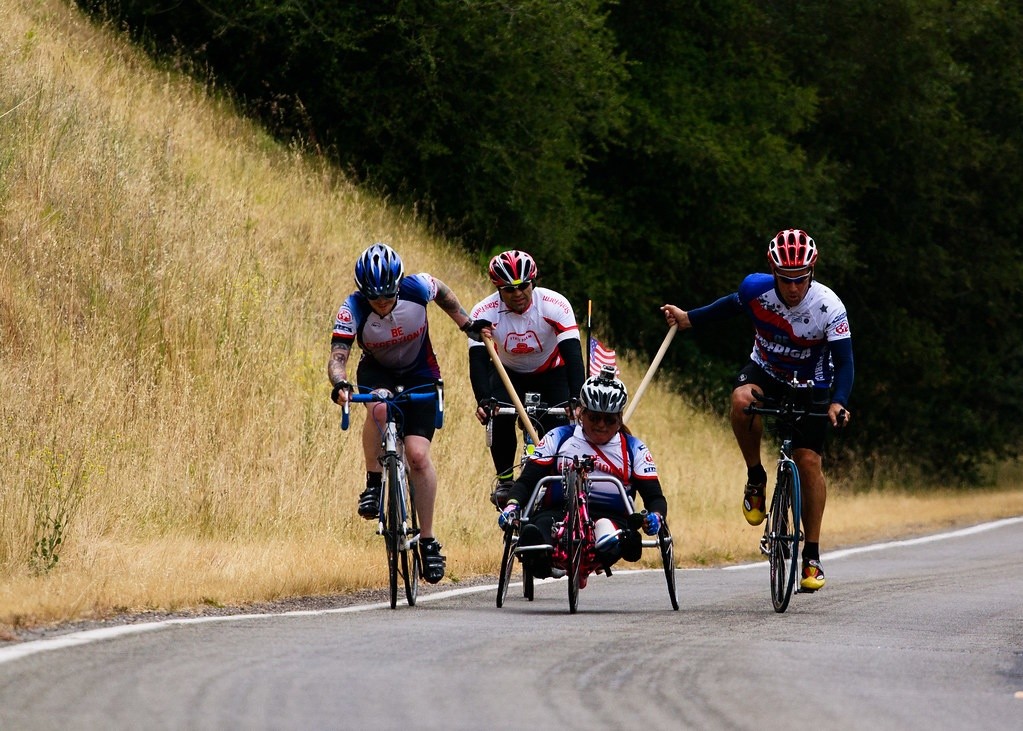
[497,465,678,614]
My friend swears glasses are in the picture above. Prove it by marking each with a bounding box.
[774,270,812,284]
[503,282,530,293]
[366,293,395,300]
[584,409,619,425]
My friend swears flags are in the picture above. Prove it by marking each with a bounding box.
[589,337,620,380]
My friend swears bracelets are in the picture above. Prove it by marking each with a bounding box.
[461,319,473,332]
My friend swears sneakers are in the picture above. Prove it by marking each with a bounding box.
[357,482,382,517]
[490,478,515,508]
[416,536,446,584]
[800,556,826,588]
[742,470,768,526]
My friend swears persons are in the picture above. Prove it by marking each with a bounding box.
[328,243,496,584]
[498,375,667,579]
[466,250,588,506]
[661,227,853,590]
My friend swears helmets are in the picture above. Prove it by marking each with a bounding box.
[580,374,629,413]
[354,242,405,296]
[488,250,538,290]
[767,228,818,277]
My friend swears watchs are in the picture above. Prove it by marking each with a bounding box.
[655,513,665,526]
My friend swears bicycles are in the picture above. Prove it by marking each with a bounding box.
[482,402,574,602]
[742,394,844,612]
[339,380,445,607]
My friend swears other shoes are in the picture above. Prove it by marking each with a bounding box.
[522,524,554,579]
